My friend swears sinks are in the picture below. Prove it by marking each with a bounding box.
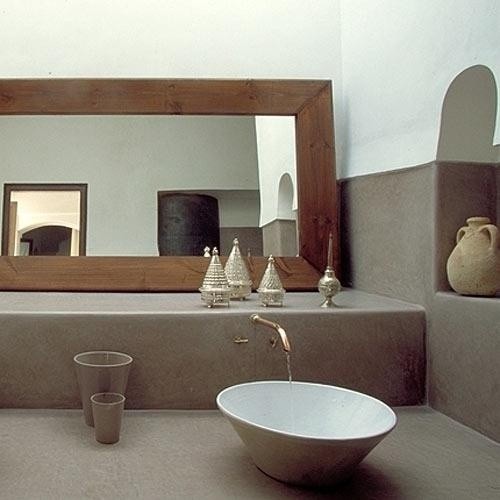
[215,379,398,488]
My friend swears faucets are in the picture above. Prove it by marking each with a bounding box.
[249,314,293,354]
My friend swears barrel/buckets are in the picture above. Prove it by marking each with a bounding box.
[157,192,221,257]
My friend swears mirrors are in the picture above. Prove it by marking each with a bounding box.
[0,76,341,292]
